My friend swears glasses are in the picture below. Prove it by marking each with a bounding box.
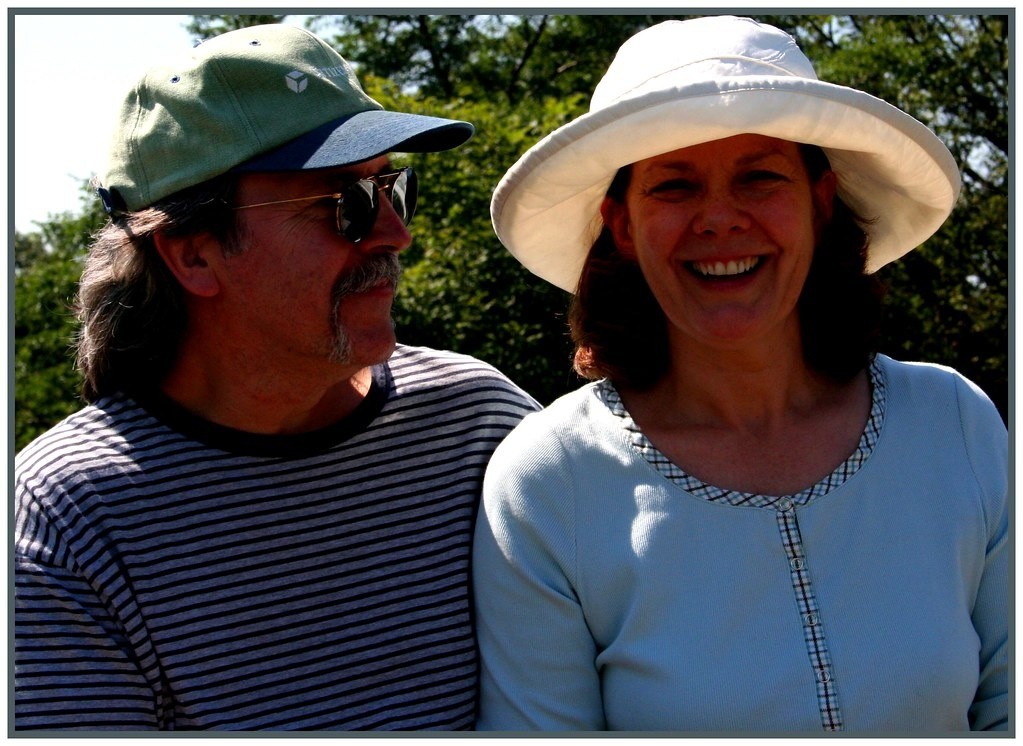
[196,167,419,243]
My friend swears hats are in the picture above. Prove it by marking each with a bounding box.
[489,15,961,296]
[96,24,475,224]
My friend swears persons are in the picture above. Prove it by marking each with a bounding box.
[13,19,544,733]
[474,14,1014,738]
